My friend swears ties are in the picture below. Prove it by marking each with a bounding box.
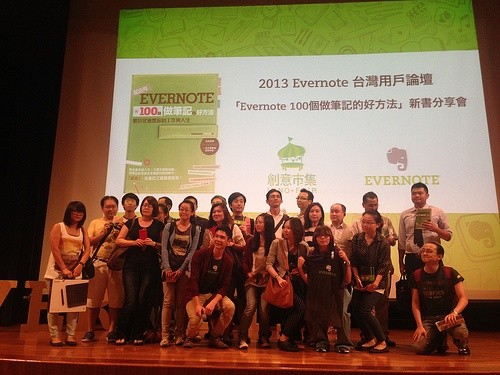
[413,221,425,248]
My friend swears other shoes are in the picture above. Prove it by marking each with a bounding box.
[338,347,349,353]
[259,336,271,347]
[370,345,389,353]
[81,331,94,342]
[308,341,315,347]
[65,336,77,346]
[356,342,379,351]
[107,332,161,345]
[183,337,194,347]
[209,338,228,348]
[175,337,184,344]
[161,339,169,346]
[386,338,395,346]
[458,343,471,355]
[51,338,63,346]
[315,347,326,351]
[278,339,298,352]
[239,339,248,349]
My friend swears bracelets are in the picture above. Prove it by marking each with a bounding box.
[275,275,279,279]
[344,261,351,266]
[453,310,459,314]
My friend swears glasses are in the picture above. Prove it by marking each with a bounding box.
[317,234,330,238]
[360,219,378,226]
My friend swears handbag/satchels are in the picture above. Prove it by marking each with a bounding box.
[108,217,137,270]
[262,274,294,308]
[230,224,244,267]
[80,250,96,280]
[395,272,408,310]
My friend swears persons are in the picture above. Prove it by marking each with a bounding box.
[44,182,472,356]
